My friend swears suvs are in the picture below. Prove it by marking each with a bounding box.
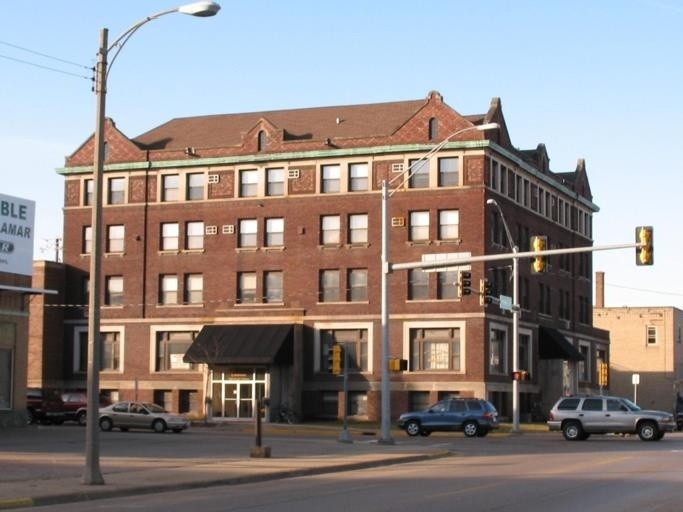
[60,391,110,424]
[26,386,65,426]
[397,396,499,438]
[546,394,678,442]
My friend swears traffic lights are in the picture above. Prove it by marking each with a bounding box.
[327,345,341,376]
[510,372,520,380]
[479,278,492,307]
[389,359,407,372]
[457,270,470,297]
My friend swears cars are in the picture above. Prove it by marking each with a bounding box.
[98,400,190,433]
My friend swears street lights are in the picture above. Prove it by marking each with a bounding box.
[486,198,523,434]
[78,2,221,485]
[376,123,502,444]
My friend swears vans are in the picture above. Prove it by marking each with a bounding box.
[672,396,683,432]
